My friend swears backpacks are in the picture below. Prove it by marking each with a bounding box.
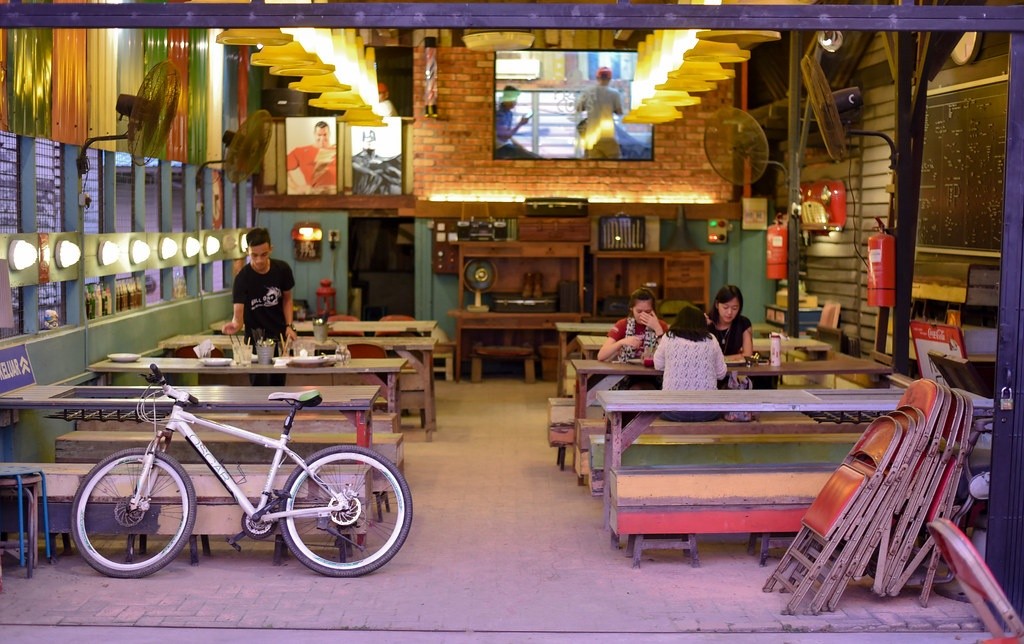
[725,371,755,423]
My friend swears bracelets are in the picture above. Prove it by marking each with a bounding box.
[655,331,666,339]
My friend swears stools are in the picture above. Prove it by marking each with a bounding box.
[0,462,52,582]
[470,343,536,380]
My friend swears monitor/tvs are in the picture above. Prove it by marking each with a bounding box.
[493,48,655,161]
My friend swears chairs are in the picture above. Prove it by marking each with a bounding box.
[762,376,1024,644]
[374,314,420,337]
[349,341,389,359]
[326,316,364,336]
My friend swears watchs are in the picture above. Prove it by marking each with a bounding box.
[286,324,296,331]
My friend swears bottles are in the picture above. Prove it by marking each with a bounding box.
[770,335,782,367]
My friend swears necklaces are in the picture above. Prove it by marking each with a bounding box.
[715,323,731,345]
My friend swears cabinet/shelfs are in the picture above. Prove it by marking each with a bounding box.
[592,249,718,317]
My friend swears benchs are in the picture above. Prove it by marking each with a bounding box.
[607,463,837,562]
[590,432,859,504]
[433,340,455,381]
[542,395,610,473]
[554,355,577,396]
[75,403,402,432]
[52,430,405,524]
[0,461,376,573]
[575,414,880,485]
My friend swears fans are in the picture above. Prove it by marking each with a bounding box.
[796,53,901,181]
[704,106,789,189]
[195,109,274,192]
[75,59,181,176]
[466,256,501,311]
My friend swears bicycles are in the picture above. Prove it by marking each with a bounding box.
[70,364,413,579]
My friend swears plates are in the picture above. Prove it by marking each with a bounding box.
[271,356,337,367]
[198,357,232,366]
[107,353,140,362]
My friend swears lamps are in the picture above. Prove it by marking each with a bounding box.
[621,0,818,128]
[815,27,852,53]
[129,239,153,265]
[203,235,221,256]
[52,238,84,268]
[10,238,39,271]
[158,236,178,260]
[97,240,121,268]
[218,27,389,131]
[423,36,438,118]
[238,234,249,252]
[183,236,201,258]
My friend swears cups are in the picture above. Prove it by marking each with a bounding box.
[231,344,253,367]
[255,345,276,365]
[277,342,291,356]
[313,325,328,343]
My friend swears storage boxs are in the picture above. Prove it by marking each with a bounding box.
[598,215,646,249]
[600,292,630,315]
[261,84,310,120]
[516,215,592,242]
[523,195,590,215]
[538,336,561,381]
[558,279,578,310]
[776,290,821,308]
[491,292,557,312]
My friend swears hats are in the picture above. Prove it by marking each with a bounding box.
[596,67,612,79]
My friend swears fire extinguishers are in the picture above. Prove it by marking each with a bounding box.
[867,216,896,307]
[766,213,788,279]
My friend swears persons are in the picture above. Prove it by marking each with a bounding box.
[379,82,399,117]
[653,304,728,423]
[222,228,298,386]
[285,121,337,194]
[494,86,539,159]
[598,288,668,418]
[704,284,754,363]
[577,66,622,160]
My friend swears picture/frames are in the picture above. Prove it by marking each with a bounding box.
[281,117,339,196]
[349,117,404,196]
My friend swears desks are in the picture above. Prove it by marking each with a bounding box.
[576,332,828,359]
[87,350,409,413]
[595,390,991,553]
[568,356,895,473]
[157,333,440,444]
[450,239,586,315]
[0,383,383,548]
[448,311,591,383]
[208,317,440,336]
[553,319,783,397]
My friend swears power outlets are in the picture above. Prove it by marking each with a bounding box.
[79,193,91,206]
[196,202,203,213]
[328,228,340,243]
